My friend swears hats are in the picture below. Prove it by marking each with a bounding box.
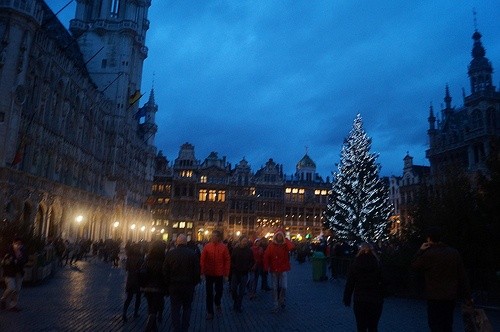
[253,238,260,244]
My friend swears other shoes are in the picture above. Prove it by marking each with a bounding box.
[261,287,272,291]
[234,306,242,313]
[208,311,213,320]
[216,307,223,320]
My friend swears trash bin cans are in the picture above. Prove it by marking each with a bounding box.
[310,255,329,282]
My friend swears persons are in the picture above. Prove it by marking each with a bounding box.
[22,235,152,268]
[199,229,230,321]
[342,243,390,332]
[291,239,332,264]
[186,238,208,255]
[162,233,203,332]
[228,234,257,314]
[246,239,264,299]
[121,242,150,322]
[262,229,294,314]
[140,239,168,331]
[0,239,29,311]
[410,225,475,332]
[223,239,241,255]
[258,237,271,292]
